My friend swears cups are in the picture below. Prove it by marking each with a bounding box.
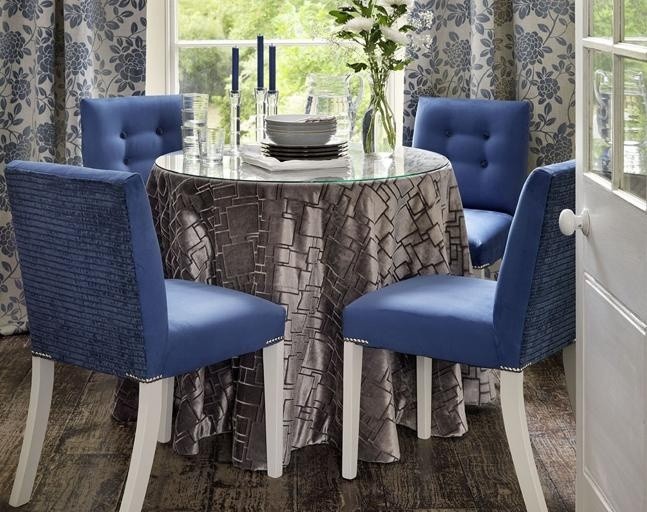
[177,91,230,165]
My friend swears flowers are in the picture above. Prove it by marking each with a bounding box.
[324,0,424,152]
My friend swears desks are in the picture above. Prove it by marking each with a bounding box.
[145,141,470,471]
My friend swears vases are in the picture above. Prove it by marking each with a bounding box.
[358,80,395,156]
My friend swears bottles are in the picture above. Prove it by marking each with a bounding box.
[228,89,240,155]
[265,89,278,116]
[254,86,267,144]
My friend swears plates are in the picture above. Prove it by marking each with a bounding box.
[260,137,351,161]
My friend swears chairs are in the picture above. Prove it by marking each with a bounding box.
[76,92,195,180]
[412,97,534,277]
[333,159,587,511]
[4,157,291,511]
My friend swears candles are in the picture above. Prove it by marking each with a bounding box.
[255,33,264,90]
[270,43,277,91]
[232,42,238,90]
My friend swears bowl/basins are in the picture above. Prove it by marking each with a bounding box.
[264,113,337,146]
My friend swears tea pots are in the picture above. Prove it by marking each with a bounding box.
[304,73,365,140]
